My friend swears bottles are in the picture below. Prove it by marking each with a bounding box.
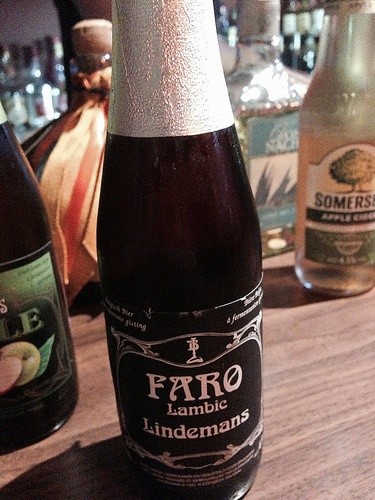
[96,0,263,500]
[0,20,68,140]
[294,0,375,297]
[0,98,80,453]
[224,0,314,269]
